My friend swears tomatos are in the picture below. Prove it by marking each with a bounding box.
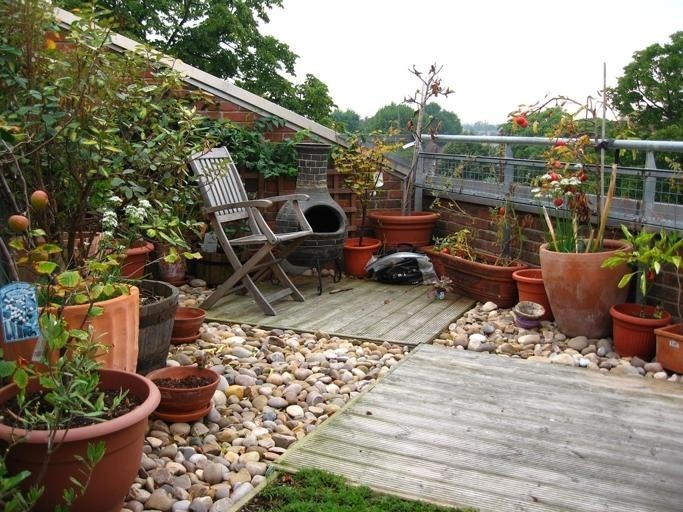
[550,174,558,181]
[556,140,564,147]
[553,199,563,206]
[499,208,505,214]
[553,161,560,168]
[443,247,449,254]
[515,117,528,128]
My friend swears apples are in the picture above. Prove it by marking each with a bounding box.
[10,216,28,230]
[31,191,48,209]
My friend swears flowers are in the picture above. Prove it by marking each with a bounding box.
[507,88,638,252]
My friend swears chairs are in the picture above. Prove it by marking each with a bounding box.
[184,147,315,316]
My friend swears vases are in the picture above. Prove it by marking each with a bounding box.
[539,239,636,339]
[512,268,554,323]
[513,301,546,329]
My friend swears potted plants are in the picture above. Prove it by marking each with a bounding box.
[600,221,682,378]
[417,140,536,311]
[2,1,238,509]
[367,62,456,255]
[331,119,406,277]
